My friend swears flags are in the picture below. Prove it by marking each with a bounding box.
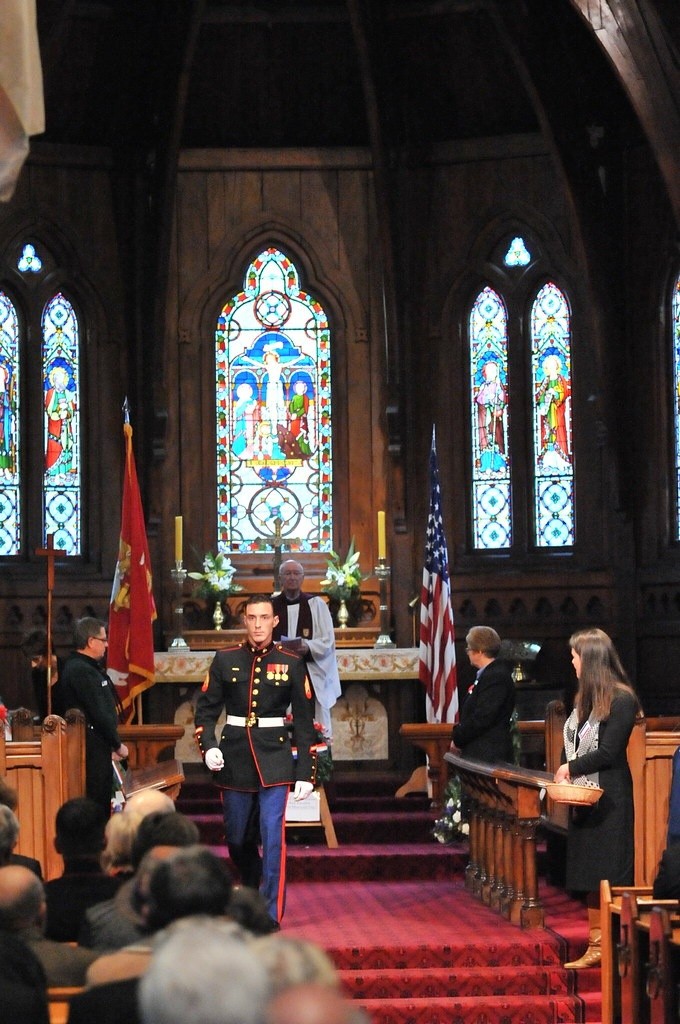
[105,422,159,727]
[418,436,461,773]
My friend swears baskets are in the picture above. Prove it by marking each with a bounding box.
[545,783,603,805]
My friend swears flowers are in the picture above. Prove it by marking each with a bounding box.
[428,773,469,845]
[187,546,244,602]
[319,536,361,601]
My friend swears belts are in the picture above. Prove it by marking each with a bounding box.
[226,714,285,727]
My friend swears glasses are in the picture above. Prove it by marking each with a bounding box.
[91,637,107,643]
[280,571,304,577]
[466,647,477,653]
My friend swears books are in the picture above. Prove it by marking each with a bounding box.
[282,637,302,651]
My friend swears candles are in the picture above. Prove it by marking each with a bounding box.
[378,510,386,558]
[175,516,183,561]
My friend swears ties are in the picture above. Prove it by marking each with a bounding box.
[99,665,126,723]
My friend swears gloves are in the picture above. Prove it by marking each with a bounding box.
[204,747,224,771]
[293,781,314,802]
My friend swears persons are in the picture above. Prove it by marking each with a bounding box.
[270,560,342,763]
[554,627,646,968]
[0,789,370,1024]
[449,627,518,768]
[21,617,127,815]
[191,595,317,933]
[652,749,680,906]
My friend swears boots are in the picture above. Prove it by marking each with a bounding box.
[564,908,603,969]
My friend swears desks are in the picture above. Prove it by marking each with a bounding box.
[33,724,185,800]
[399,716,680,812]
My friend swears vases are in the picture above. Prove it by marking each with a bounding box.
[336,599,349,628]
[213,601,224,630]
